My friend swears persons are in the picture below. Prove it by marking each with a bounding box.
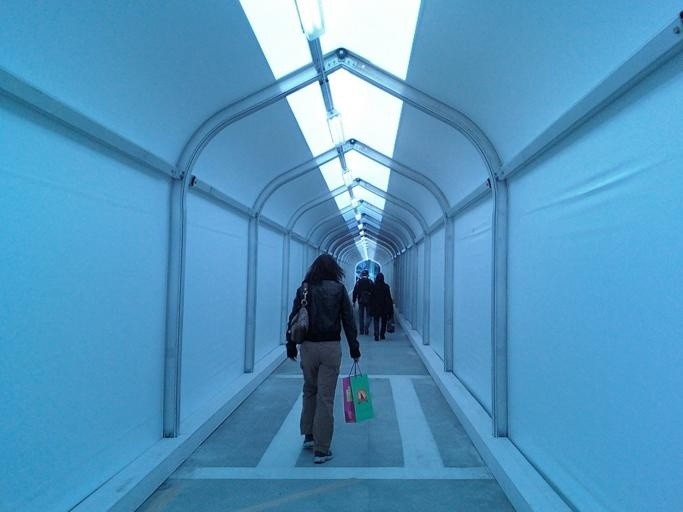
[285,255,361,463]
[352,270,373,335]
[365,273,393,341]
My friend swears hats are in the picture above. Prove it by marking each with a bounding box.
[362,270,369,276]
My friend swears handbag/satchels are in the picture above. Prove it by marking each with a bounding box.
[387,318,396,333]
[287,295,310,343]
[343,372,374,424]
[358,290,371,305]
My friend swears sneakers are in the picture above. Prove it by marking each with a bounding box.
[303,433,313,448]
[314,450,333,463]
[360,326,385,340]
